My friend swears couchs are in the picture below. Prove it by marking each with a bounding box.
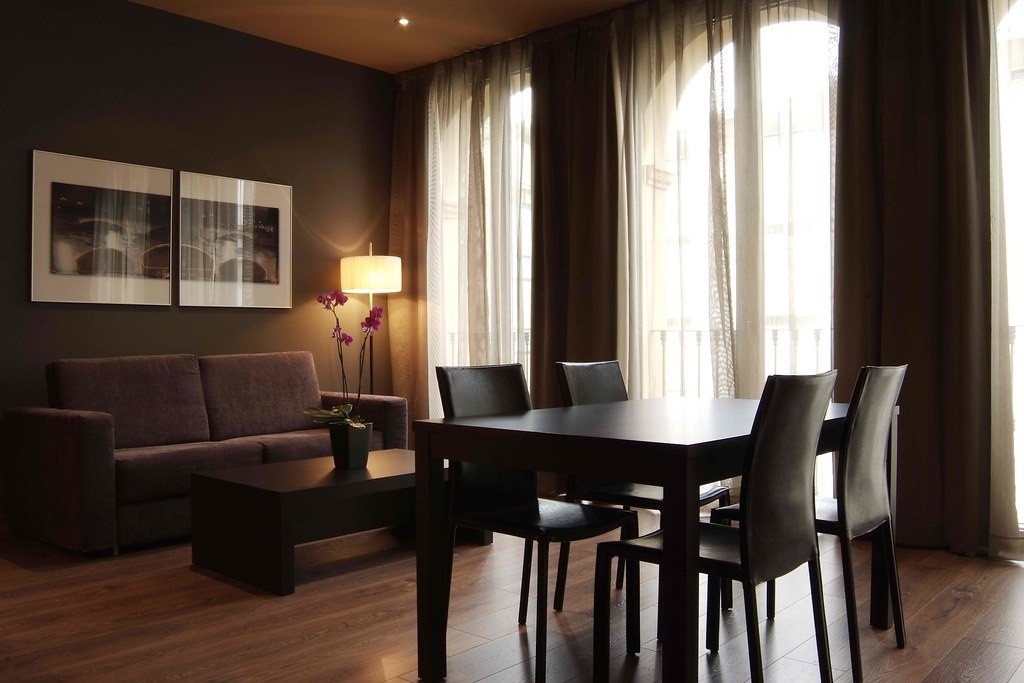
[2,350,408,556]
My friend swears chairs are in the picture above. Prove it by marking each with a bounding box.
[707,363,907,683]
[593,369,838,683]
[436,364,640,683]
[555,360,734,612]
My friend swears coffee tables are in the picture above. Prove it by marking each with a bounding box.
[190,449,449,596]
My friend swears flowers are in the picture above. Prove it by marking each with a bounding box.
[303,289,383,430]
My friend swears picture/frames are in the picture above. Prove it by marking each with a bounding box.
[31,149,173,307]
[179,171,293,309]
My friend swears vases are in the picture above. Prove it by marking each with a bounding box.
[329,423,373,475]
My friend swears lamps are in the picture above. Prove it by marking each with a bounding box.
[340,242,402,395]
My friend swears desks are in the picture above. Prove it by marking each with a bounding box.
[412,397,900,683]
